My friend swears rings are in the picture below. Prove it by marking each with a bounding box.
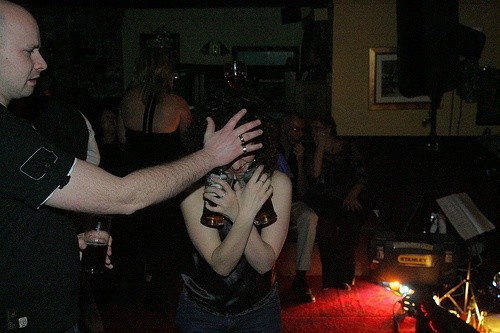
[259,179,265,184]
[239,134,246,144]
[243,145,248,154]
[219,191,226,198]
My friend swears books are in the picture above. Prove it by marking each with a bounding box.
[436,192,496,240]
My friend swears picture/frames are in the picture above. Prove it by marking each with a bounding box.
[368,47,444,111]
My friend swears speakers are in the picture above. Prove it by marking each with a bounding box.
[370,232,457,292]
[412,290,480,333]
[395,0,459,98]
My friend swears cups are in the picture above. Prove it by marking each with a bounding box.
[78,215,111,273]
[199,169,233,230]
[236,162,277,229]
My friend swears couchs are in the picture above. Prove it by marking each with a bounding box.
[339,135,500,234]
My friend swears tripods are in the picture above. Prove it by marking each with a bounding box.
[435,193,496,329]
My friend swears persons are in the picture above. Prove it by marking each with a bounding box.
[4,47,372,333]
[0,0,263,333]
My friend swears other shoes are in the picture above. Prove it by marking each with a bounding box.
[323,280,351,293]
[291,283,316,303]
